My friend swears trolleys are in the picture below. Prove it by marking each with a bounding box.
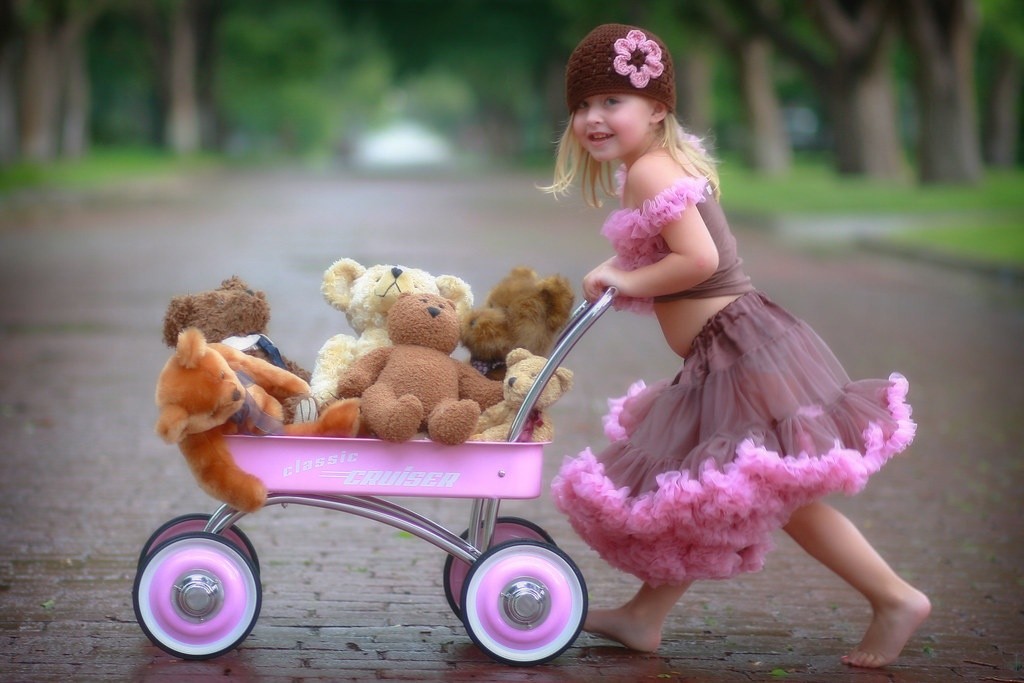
[131,284,621,668]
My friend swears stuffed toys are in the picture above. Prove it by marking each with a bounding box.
[310,256,475,397]
[459,267,575,381]
[467,348,574,444]
[161,274,312,425]
[155,326,360,513]
[336,290,503,446]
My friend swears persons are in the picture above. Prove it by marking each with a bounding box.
[534,22,932,669]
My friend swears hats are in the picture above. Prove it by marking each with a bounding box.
[565,24,674,115]
